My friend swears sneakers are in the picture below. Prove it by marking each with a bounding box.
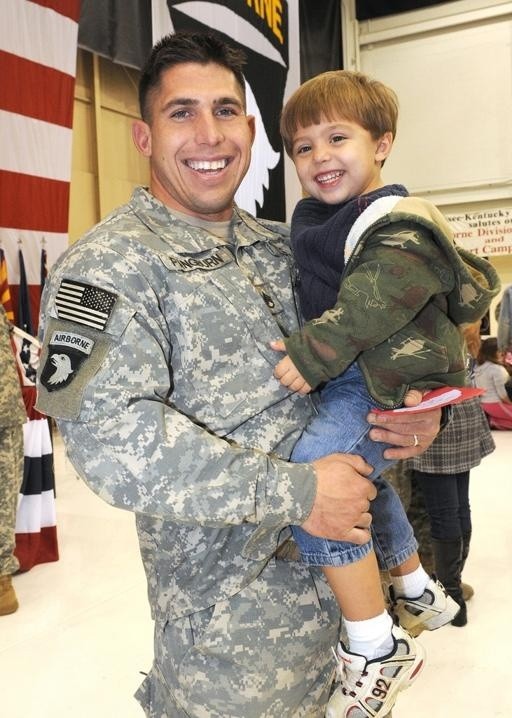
[325,622,423,718]
[386,580,473,639]
[0,576,18,616]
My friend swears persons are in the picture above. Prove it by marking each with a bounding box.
[403,282,510,632]
[267,70,501,717]
[31,29,454,718]
[2,304,22,617]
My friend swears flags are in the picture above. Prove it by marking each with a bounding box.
[131,0,303,227]
[42,241,49,297]
[1,0,83,338]
[18,243,35,338]
[1,247,16,326]
[8,326,61,575]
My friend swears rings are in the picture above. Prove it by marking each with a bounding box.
[410,434,418,448]
